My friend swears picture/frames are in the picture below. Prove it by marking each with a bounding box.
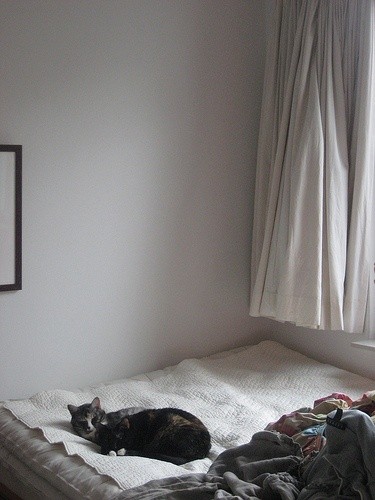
[0,144,23,292]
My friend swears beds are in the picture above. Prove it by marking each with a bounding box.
[0,338,375,500]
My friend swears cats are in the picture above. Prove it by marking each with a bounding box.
[66,396,213,467]
